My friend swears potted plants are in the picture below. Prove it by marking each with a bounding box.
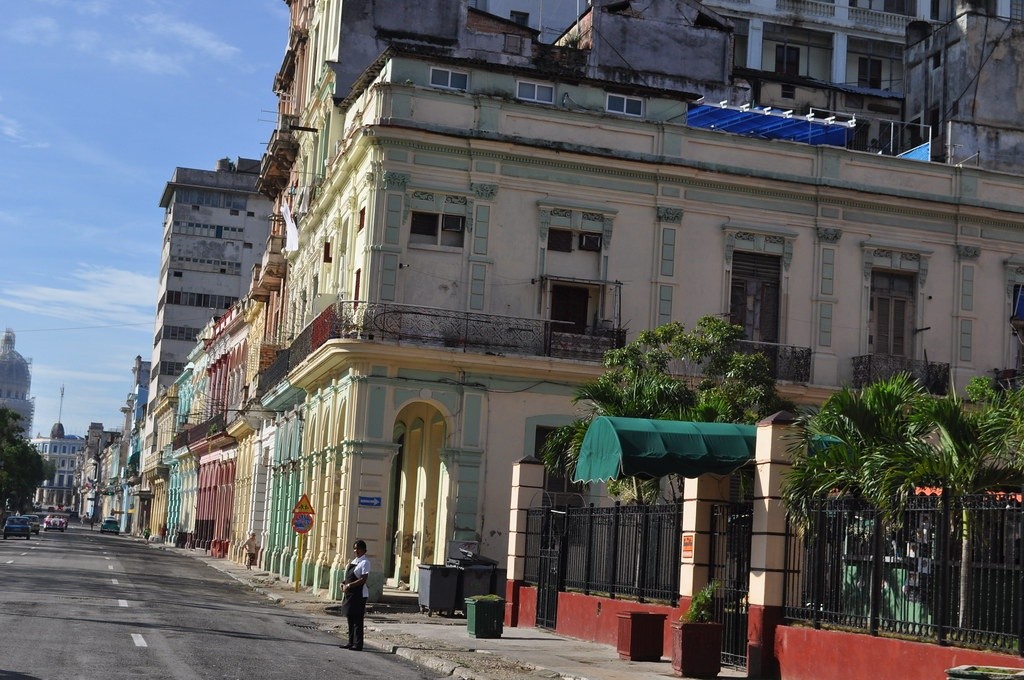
[670,581,725,678]
[463,594,507,638]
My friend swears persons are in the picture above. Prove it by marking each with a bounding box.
[243,531,256,571]
[339,541,371,650]
[81,515,86,527]
[160,523,168,540]
[90,515,96,531]
[16,510,20,517]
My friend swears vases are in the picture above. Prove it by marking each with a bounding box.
[944,664,1024,680]
[617,610,667,661]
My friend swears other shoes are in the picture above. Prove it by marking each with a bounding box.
[340,643,363,651]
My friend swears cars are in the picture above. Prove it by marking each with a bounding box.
[22,515,40,535]
[48,507,55,513]
[3,516,33,540]
[44,514,68,532]
[100,517,120,535]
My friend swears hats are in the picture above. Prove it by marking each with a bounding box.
[250,532,256,536]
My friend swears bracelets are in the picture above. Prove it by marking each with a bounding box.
[347,584,351,588]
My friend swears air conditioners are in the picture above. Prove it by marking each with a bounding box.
[443,216,462,232]
[580,235,601,251]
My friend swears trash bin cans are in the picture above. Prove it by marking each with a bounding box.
[458,548,507,601]
[416,563,459,617]
[446,557,493,617]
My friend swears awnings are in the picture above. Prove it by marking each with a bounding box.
[569,416,844,485]
